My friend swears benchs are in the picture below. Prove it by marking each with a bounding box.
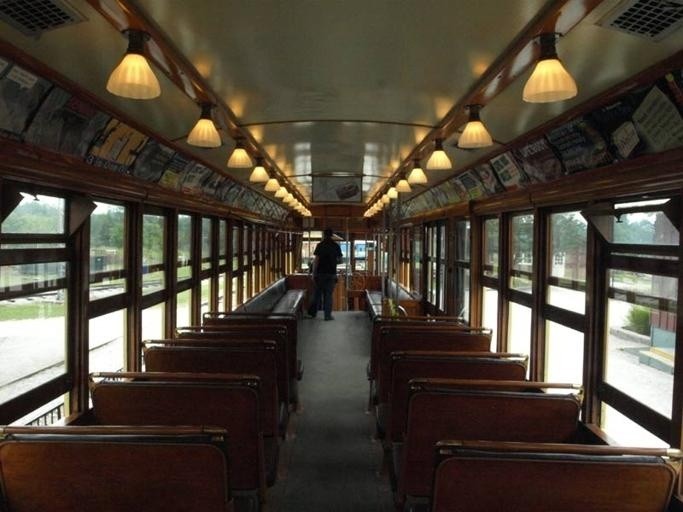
[365,316,682,510]
[1,311,307,510]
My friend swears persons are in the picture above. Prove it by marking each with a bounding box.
[307,227,343,320]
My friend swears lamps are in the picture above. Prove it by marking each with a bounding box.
[106,26,161,100]
[188,100,222,148]
[426,138,453,170]
[226,136,253,168]
[362,190,390,218]
[458,104,493,148]
[250,156,269,183]
[522,32,578,103]
[388,181,398,198]
[263,172,279,190]
[407,158,427,184]
[394,172,411,192]
[281,192,312,218]
[274,181,287,197]
